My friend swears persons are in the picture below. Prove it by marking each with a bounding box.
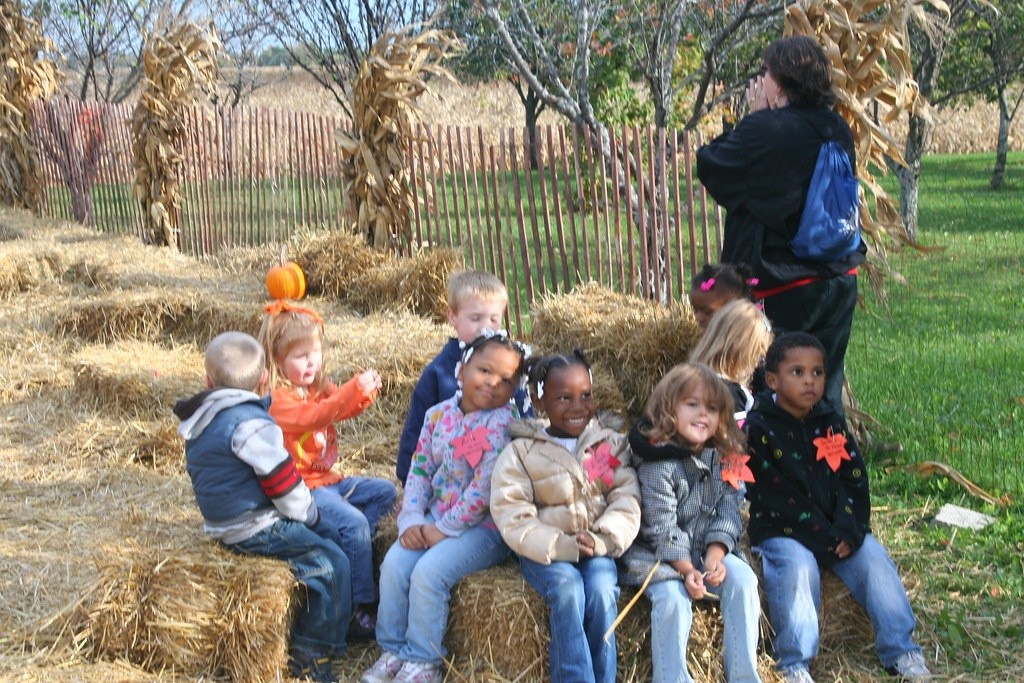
[697,37,868,421]
[363,262,936,683]
[174,302,395,683]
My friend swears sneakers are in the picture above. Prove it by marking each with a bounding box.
[358,652,403,683]
[390,661,442,683]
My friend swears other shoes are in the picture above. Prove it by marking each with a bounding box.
[287,649,338,683]
[884,649,932,683]
[346,608,375,644]
[776,665,815,683]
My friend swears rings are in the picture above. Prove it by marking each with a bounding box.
[750,97,755,101]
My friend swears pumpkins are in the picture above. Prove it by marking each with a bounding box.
[267,244,306,300]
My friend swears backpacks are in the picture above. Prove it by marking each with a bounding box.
[760,107,861,262]
